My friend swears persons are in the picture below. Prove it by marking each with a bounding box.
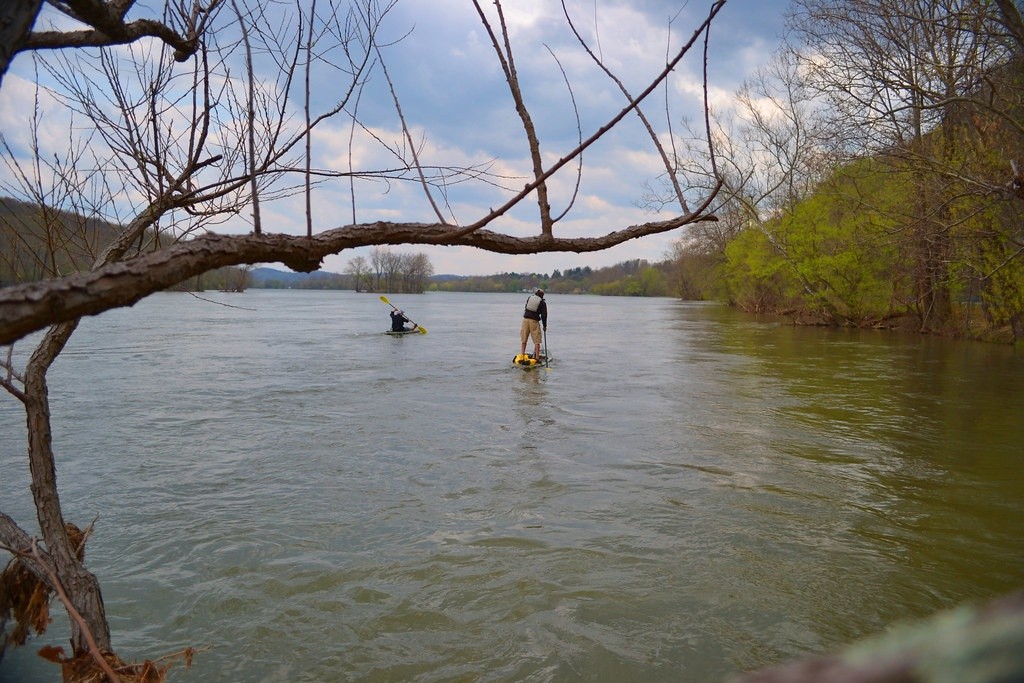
[390,308,418,332]
[519,289,547,360]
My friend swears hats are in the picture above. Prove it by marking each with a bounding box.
[536,289,544,295]
[397,310,403,314]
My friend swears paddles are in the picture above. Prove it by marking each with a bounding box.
[544,330,551,370]
[380,296,428,334]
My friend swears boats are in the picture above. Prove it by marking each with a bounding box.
[384,330,420,335]
[513,345,553,370]
